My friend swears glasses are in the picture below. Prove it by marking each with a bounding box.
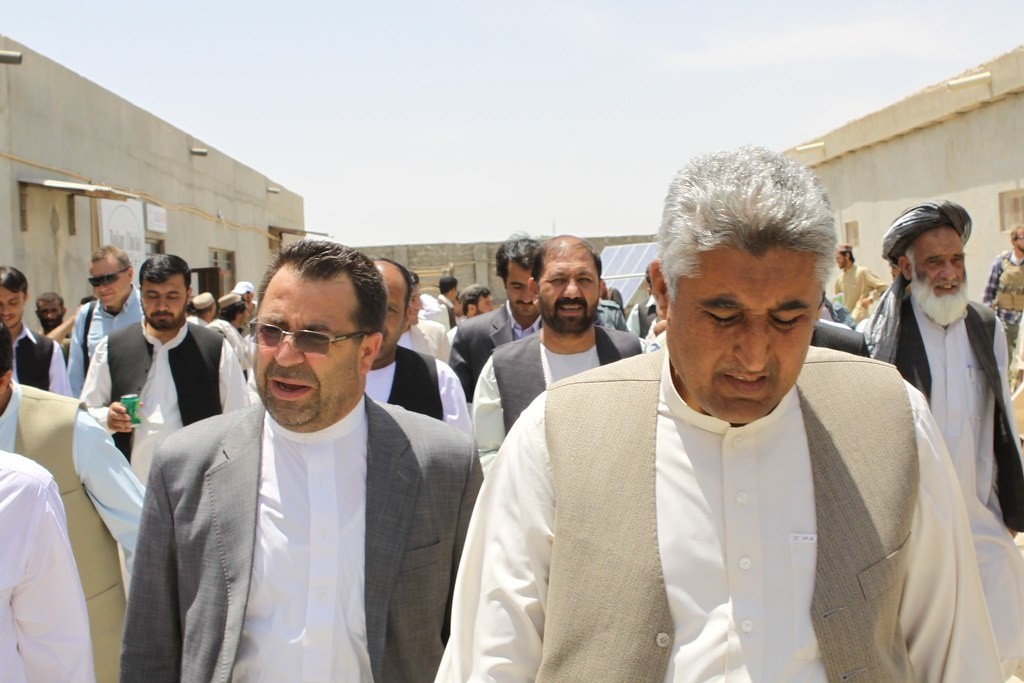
[88,267,128,286]
[246,318,372,354]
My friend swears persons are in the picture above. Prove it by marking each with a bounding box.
[81,254,250,500]
[834,245,886,328]
[66,246,148,400]
[119,240,484,682]
[854,200,1024,683]
[435,148,1001,682]
[0,235,872,682]
[982,227,1022,374]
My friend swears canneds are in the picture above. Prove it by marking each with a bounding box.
[120,394,141,428]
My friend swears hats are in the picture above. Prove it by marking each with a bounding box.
[836,244,852,252]
[218,294,241,308]
[192,292,214,309]
[232,282,253,294]
